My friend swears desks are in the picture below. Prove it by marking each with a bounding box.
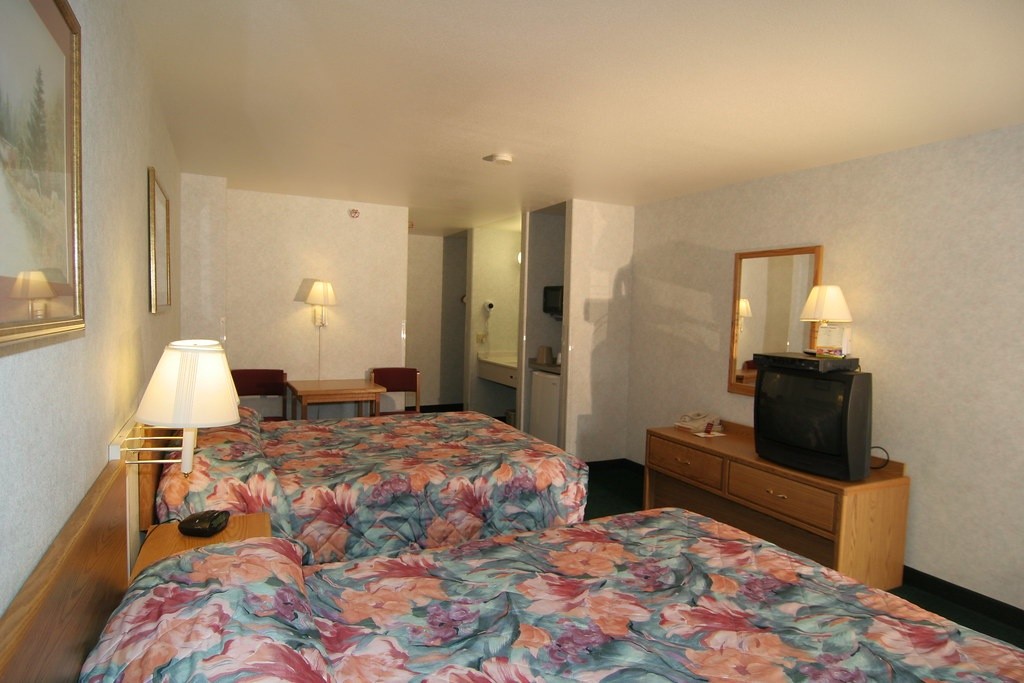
[644,419,909,591]
[289,379,387,421]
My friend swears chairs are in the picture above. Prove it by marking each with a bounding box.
[369,368,421,417]
[231,366,288,422]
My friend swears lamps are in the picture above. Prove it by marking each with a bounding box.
[136,338,240,449]
[10,270,52,318]
[304,282,334,325]
[800,285,852,355]
[738,299,751,333]
[124,343,242,467]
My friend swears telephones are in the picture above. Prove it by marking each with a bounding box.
[673,412,720,433]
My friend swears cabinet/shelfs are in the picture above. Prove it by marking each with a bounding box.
[475,199,637,463]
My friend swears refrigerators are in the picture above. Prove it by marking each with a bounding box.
[529,371,560,446]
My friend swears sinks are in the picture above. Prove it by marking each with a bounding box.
[504,361,517,366]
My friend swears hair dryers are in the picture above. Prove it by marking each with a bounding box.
[484,301,495,322]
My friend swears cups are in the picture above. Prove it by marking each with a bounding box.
[556,352,561,366]
[536,346,553,363]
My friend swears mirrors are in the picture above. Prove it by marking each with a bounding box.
[728,246,825,398]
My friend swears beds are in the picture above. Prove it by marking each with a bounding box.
[154,410,598,567]
[84,507,1023,683]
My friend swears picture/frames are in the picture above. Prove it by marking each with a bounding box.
[147,166,172,312]
[0,2,85,356]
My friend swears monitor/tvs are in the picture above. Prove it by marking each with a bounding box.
[754,367,873,482]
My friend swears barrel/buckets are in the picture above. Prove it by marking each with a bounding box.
[505,409,515,426]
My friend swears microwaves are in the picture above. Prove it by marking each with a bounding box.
[544,286,564,314]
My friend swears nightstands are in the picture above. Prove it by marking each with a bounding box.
[130,513,273,580]
